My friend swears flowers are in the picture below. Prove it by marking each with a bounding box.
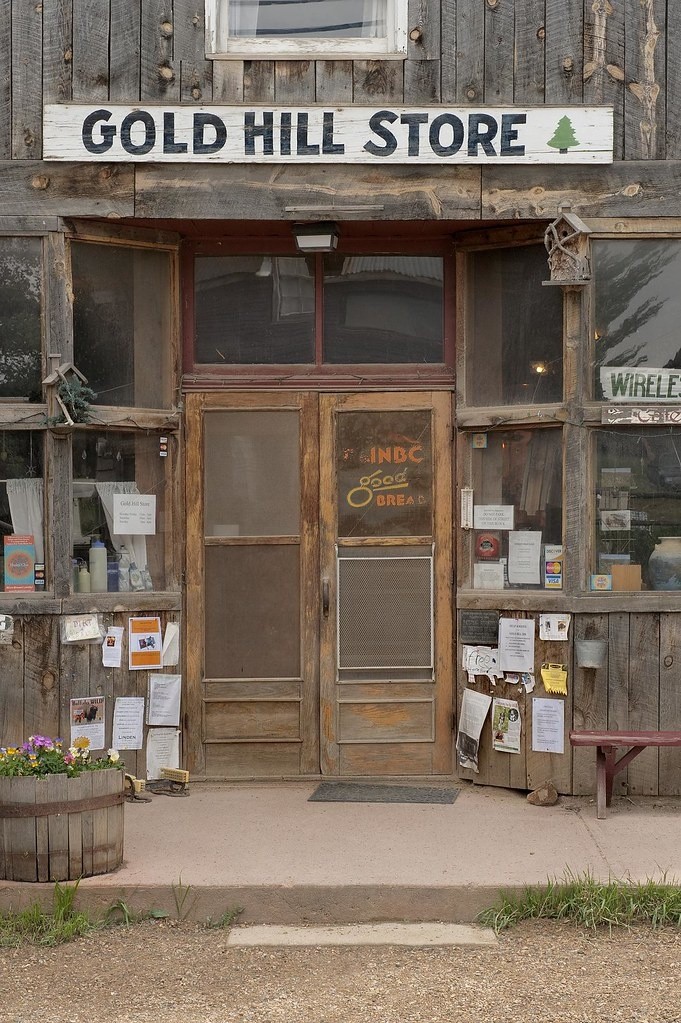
[0,734,129,780]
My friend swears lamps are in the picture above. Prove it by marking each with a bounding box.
[529,360,549,376]
[291,221,341,252]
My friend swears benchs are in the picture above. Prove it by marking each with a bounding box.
[568,730,681,820]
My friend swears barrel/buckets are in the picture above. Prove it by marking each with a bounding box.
[574,627,609,669]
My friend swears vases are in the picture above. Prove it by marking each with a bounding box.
[0,768,125,882]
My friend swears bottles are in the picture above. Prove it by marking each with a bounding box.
[71,559,89,593]
[107,544,130,591]
